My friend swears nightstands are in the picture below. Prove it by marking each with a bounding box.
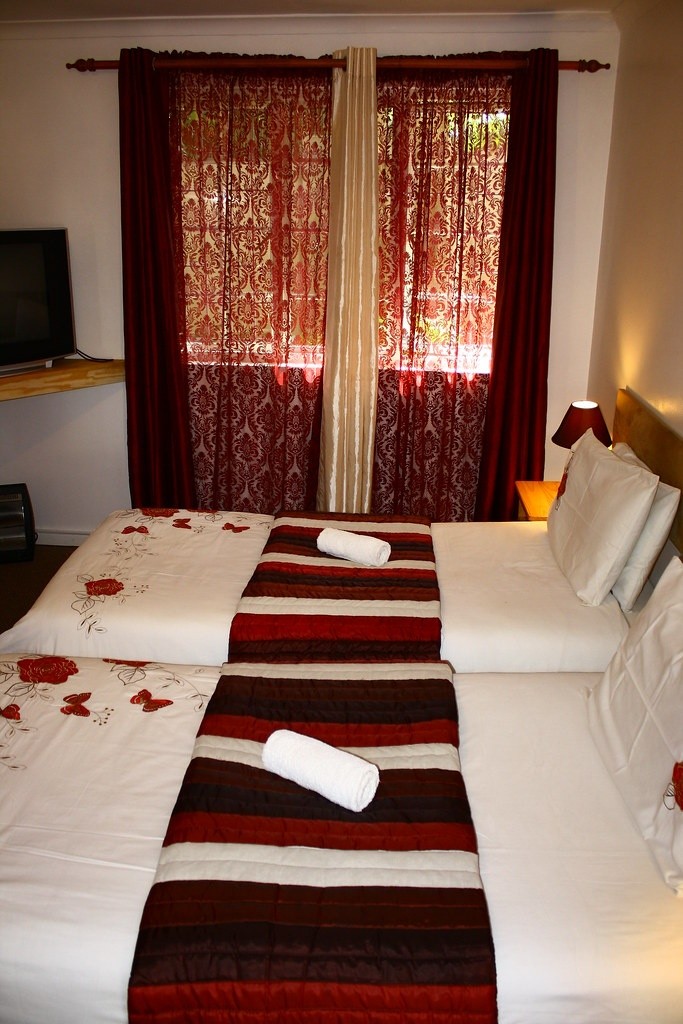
[513,480,560,521]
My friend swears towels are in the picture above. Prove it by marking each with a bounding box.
[316,527,392,567]
[262,728,380,814]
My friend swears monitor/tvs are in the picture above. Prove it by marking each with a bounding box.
[1,226,75,379]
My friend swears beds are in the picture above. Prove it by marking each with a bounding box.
[1,386,682,672]
[1,655,682,1022]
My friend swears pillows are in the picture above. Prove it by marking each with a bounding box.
[580,557,683,898]
[606,440,683,615]
[546,427,660,606]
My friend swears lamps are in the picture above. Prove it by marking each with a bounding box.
[550,399,613,449]
